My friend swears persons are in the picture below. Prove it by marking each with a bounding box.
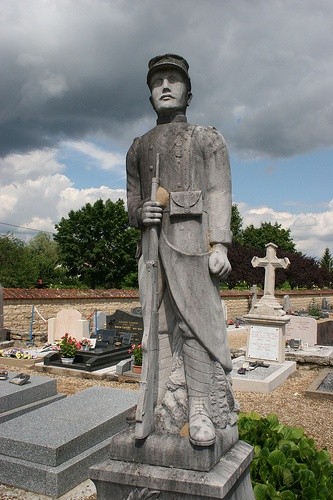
[124,53,243,442]
